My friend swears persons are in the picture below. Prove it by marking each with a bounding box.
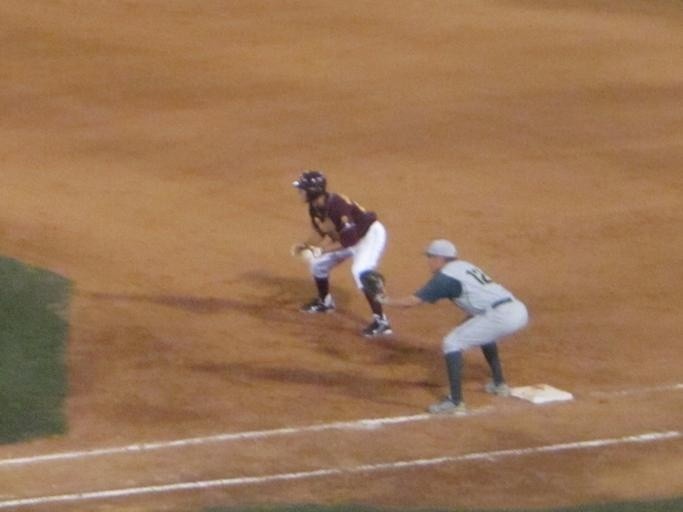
[291,171,393,338]
[376,239,530,414]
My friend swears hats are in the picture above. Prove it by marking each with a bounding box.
[418,238,457,257]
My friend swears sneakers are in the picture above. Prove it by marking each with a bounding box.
[300,294,336,313]
[427,398,467,417]
[485,382,510,397]
[361,313,394,338]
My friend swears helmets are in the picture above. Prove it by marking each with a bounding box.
[291,170,328,203]
